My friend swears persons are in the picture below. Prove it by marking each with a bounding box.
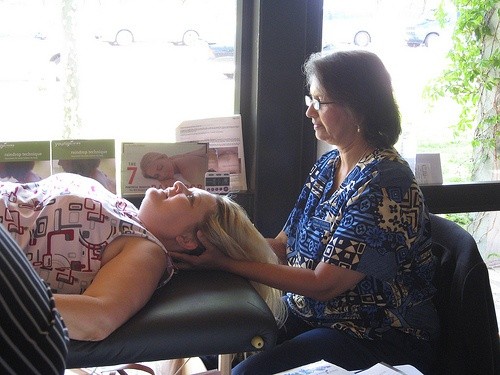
[140,146,206,188]
[0,159,116,195]
[168,51,443,375]
[0,226,70,375]
[0,173,288,341]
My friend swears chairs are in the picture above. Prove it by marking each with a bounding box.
[422,213,500,375]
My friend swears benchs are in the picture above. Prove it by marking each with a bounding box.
[64,267,277,375]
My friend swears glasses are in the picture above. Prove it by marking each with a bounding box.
[304,95,342,111]
